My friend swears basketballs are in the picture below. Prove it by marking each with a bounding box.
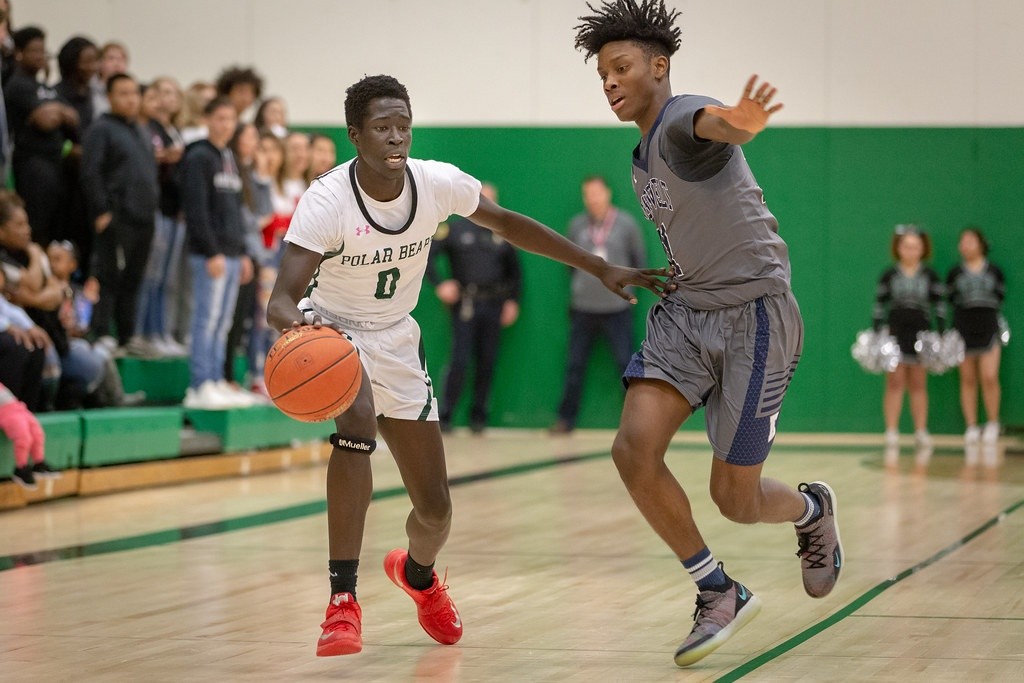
[264,325,363,425]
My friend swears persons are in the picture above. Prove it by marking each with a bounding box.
[426,183,522,438]
[266,75,676,657]
[551,176,645,436]
[0,0,336,489]
[873,224,1005,443]
[573,1,843,666]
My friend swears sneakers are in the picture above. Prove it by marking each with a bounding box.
[316,591,363,657]
[673,560,761,667]
[793,481,844,599]
[383,547,463,645]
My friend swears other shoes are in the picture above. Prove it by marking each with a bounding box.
[13,465,37,491]
[32,464,60,479]
[983,424,1000,443]
[183,378,271,410]
[128,334,187,359]
[885,429,899,444]
[914,431,932,447]
[964,427,980,443]
